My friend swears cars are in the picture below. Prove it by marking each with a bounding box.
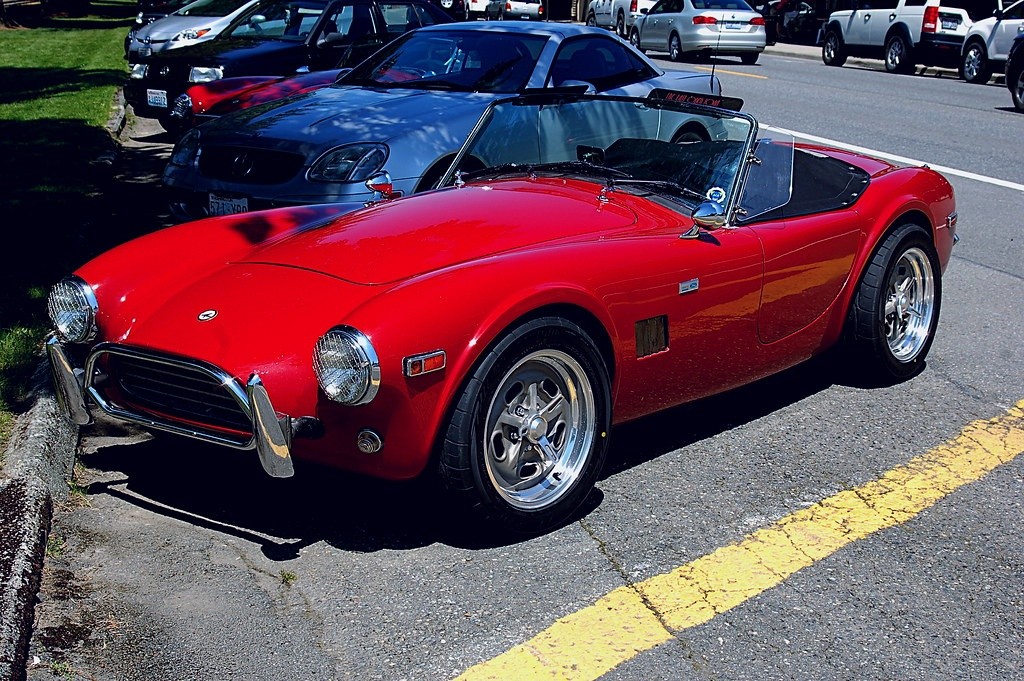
[585,0,663,39]
[960,0,1024,83]
[629,0,767,65]
[1003,21,1023,111]
[119,0,545,136]
[156,19,734,221]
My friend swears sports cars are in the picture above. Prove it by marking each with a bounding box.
[40,92,962,537]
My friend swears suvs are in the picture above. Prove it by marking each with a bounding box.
[813,1,1014,74]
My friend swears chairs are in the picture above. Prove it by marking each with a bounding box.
[480,47,522,74]
[564,49,606,79]
